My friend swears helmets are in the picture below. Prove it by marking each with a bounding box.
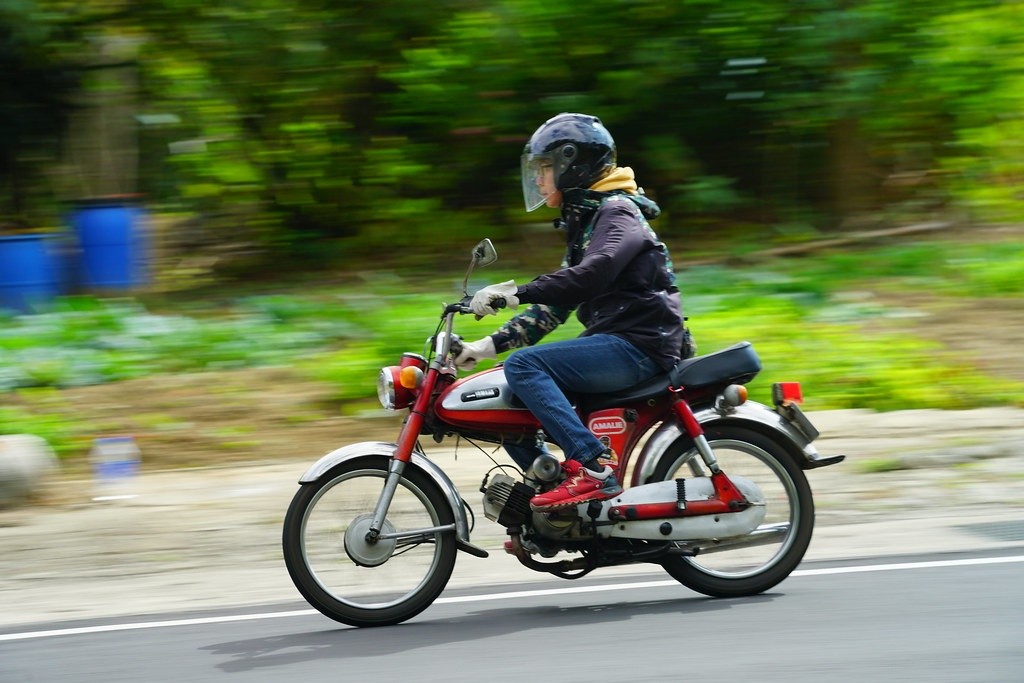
[525,112,617,192]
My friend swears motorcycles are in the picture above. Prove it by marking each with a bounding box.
[282,237,847,628]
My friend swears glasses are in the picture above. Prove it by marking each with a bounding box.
[537,163,553,175]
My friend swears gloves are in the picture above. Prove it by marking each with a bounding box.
[469,279,520,320]
[453,336,498,371]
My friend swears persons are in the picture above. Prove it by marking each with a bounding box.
[452,111,685,557]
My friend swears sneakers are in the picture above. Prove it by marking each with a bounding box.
[530,459,624,511]
[504,541,514,555]
